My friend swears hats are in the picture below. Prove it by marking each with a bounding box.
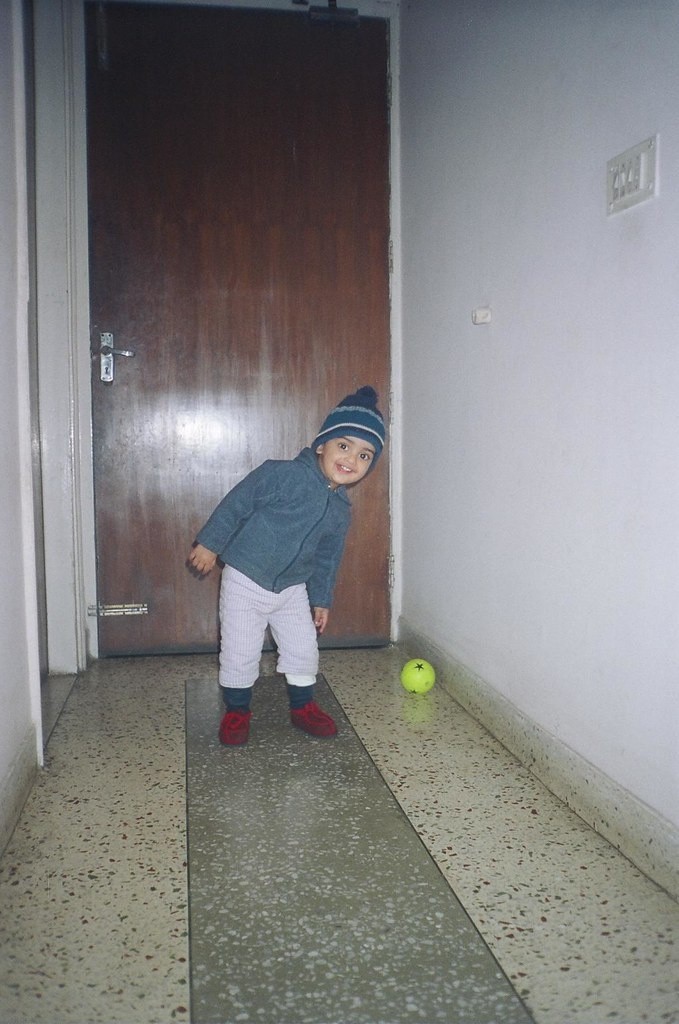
[311,385,385,475]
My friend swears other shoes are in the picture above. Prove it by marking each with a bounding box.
[291,701,337,739]
[219,710,252,746]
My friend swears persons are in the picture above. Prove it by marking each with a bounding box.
[188,385,387,746]
